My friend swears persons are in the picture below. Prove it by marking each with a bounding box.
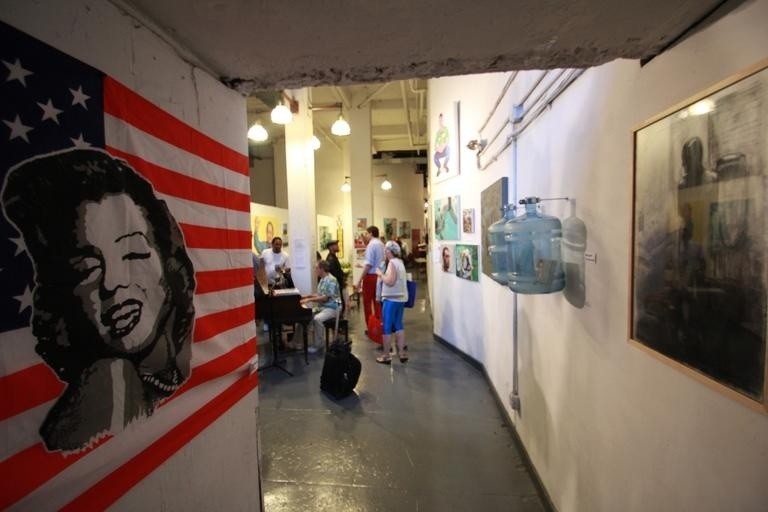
[253,216,274,253]
[253,250,260,330]
[260,237,290,332]
[677,135,721,267]
[326,241,347,333]
[3,149,197,455]
[353,226,385,335]
[317,252,321,283]
[374,241,409,364]
[300,260,341,354]
[435,197,458,243]
[432,113,452,177]
[438,247,450,273]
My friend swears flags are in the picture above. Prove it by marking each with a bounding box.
[0,20,261,510]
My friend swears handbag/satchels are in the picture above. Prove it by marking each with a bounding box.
[403,279,416,308]
[367,315,384,344]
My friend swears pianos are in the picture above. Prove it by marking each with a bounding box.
[269,288,311,366]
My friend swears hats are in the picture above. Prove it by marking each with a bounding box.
[385,239,401,256]
[327,239,339,247]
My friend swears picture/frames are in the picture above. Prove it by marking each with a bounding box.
[627,56,768,419]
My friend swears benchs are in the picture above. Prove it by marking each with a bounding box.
[308,311,349,355]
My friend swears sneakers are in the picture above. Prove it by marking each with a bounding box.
[374,345,408,352]
[285,341,304,350]
[308,344,324,353]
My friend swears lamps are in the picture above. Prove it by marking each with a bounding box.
[342,173,391,192]
[245,89,350,153]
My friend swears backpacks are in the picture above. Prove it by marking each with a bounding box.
[318,340,361,400]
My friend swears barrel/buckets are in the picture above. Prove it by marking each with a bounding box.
[560,197,587,309]
[504,198,566,295]
[486,205,534,285]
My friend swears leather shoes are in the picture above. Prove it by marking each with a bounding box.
[376,357,392,364]
[400,355,409,363]
